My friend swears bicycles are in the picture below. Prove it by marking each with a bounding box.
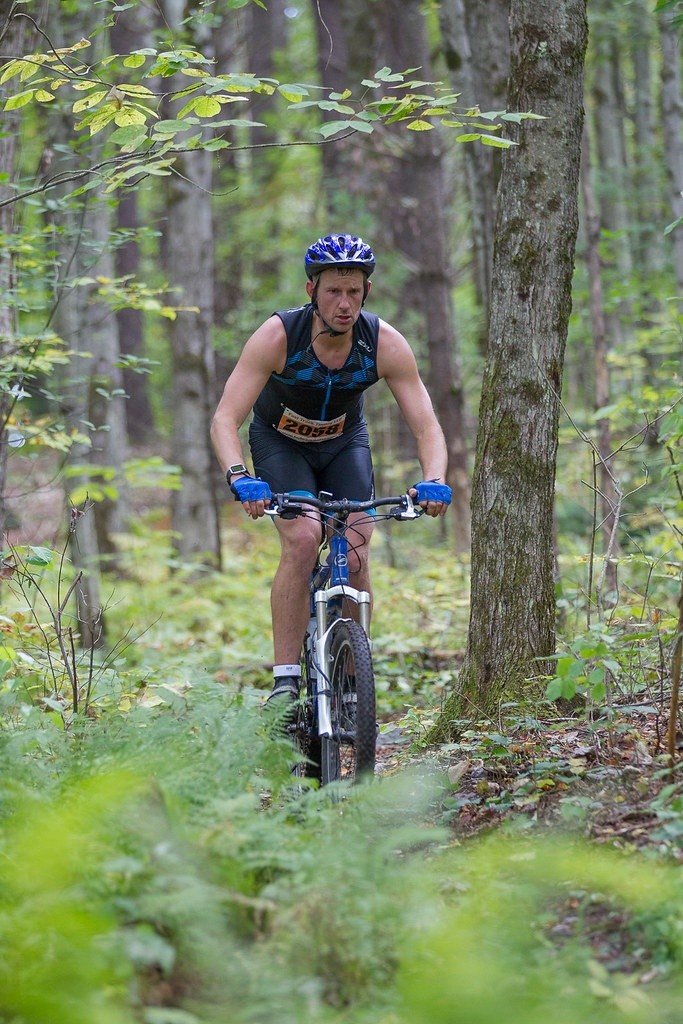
[233,491,432,807]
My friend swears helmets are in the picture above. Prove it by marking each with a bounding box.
[304,233,376,281]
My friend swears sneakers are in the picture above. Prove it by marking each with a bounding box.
[340,674,380,748]
[265,663,302,722]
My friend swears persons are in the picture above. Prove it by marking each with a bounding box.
[210,231,452,741]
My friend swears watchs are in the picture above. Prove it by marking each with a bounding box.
[226,463,250,485]
